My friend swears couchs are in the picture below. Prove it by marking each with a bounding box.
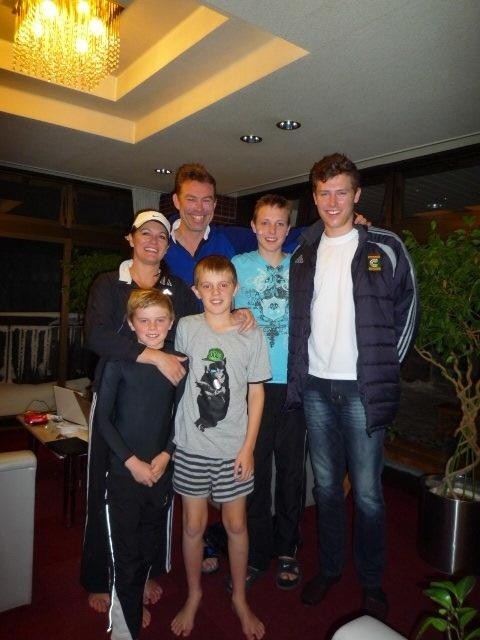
[0,375,62,419]
[1,444,44,617]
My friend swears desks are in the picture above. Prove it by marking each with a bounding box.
[16,409,88,531]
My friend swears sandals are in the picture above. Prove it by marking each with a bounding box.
[228,558,266,590]
[201,536,221,574]
[275,551,302,589]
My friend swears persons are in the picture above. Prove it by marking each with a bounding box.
[222,189,310,594]
[80,209,202,631]
[282,152,421,624]
[172,252,273,638]
[92,286,190,640]
[158,159,234,576]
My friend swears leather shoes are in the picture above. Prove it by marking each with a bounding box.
[359,572,389,622]
[300,569,343,605]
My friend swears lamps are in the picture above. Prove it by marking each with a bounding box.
[8,0,123,96]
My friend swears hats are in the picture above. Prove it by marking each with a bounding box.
[131,210,171,239]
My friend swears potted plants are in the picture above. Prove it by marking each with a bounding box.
[397,210,480,579]
